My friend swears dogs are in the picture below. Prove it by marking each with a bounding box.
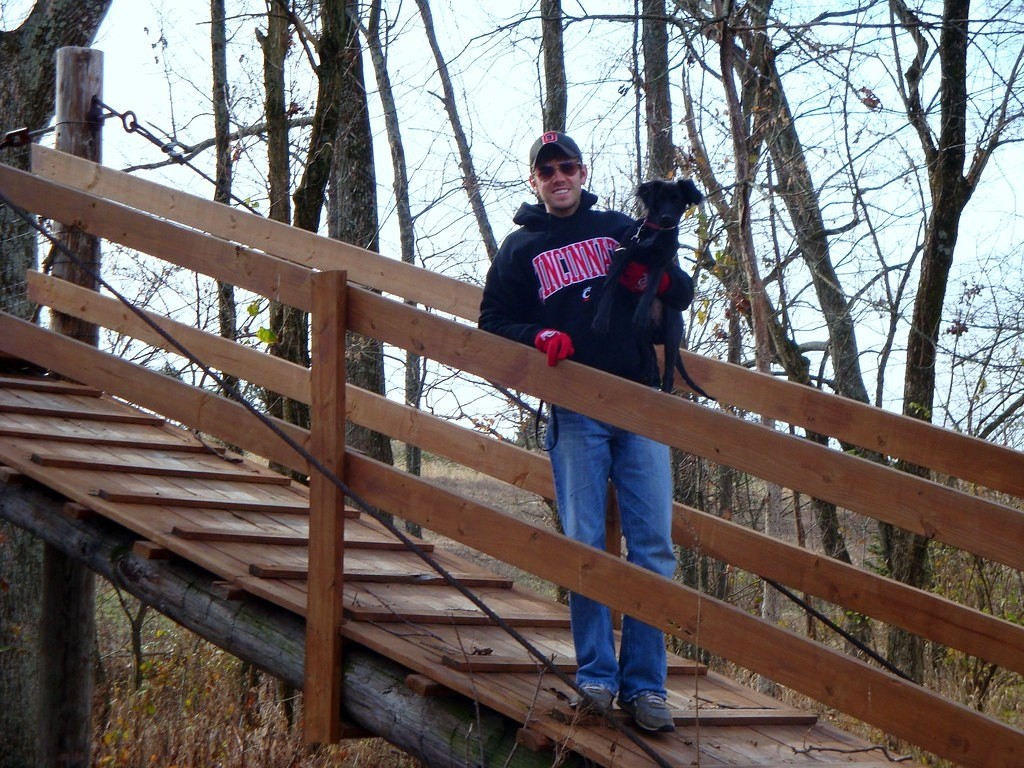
[591,180,717,401]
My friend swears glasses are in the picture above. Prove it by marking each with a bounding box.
[534,161,582,182]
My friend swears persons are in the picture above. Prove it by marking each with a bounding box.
[479,130,694,732]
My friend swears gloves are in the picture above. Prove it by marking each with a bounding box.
[617,262,670,297]
[535,328,575,367]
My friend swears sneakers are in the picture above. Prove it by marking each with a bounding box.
[616,692,675,733]
[570,682,615,714]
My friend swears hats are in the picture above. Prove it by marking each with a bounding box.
[529,131,583,173]
